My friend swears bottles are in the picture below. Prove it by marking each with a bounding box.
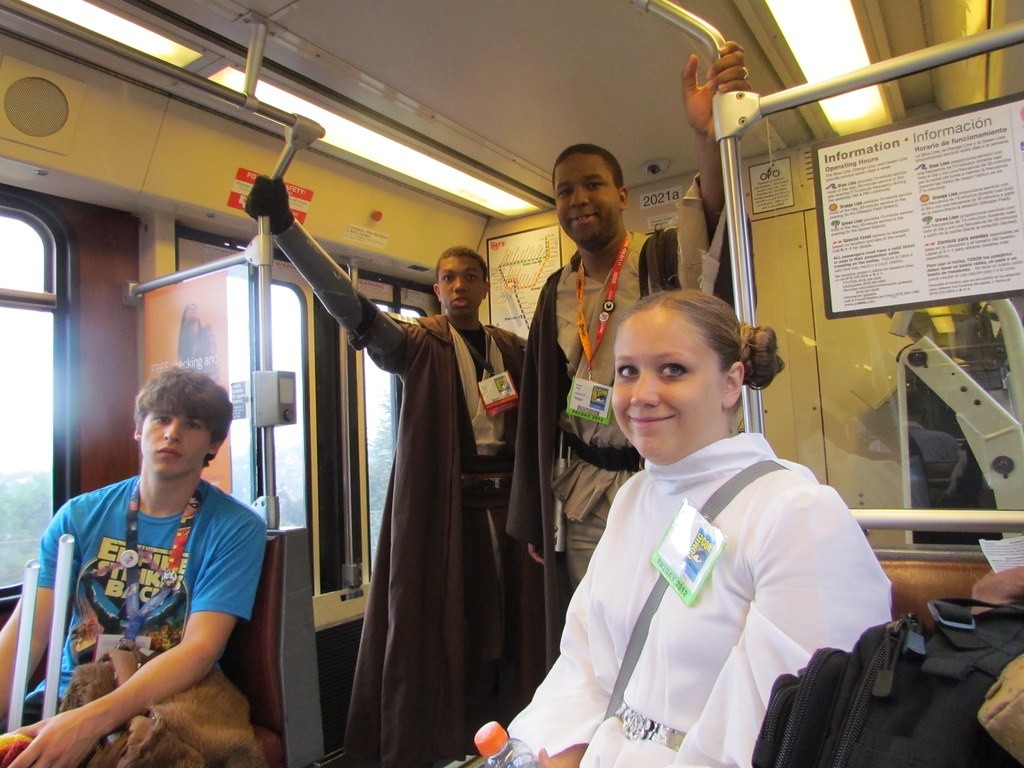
[474,721,540,768]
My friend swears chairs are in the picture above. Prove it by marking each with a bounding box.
[218,530,291,767]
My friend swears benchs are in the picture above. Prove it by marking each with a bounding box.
[848,506,1024,633]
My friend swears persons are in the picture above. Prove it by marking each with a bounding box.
[970,566,1024,615]
[0,367,269,768]
[505,40,759,675]
[907,390,993,511]
[244,174,525,768]
[508,292,893,767]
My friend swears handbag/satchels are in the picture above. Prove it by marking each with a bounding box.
[752,596,1024,768]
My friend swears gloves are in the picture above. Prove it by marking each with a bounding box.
[244,175,294,236]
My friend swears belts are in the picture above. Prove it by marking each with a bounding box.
[570,436,646,472]
